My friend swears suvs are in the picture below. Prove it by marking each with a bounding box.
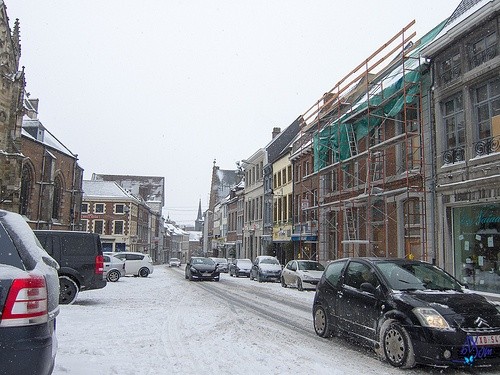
[30,228,108,306]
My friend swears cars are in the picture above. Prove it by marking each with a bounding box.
[213,258,230,273]
[184,257,220,282]
[169,257,180,267]
[229,258,254,278]
[249,256,283,283]
[102,254,126,283]
[311,256,500,371]
[280,259,326,291]
[112,252,154,277]
